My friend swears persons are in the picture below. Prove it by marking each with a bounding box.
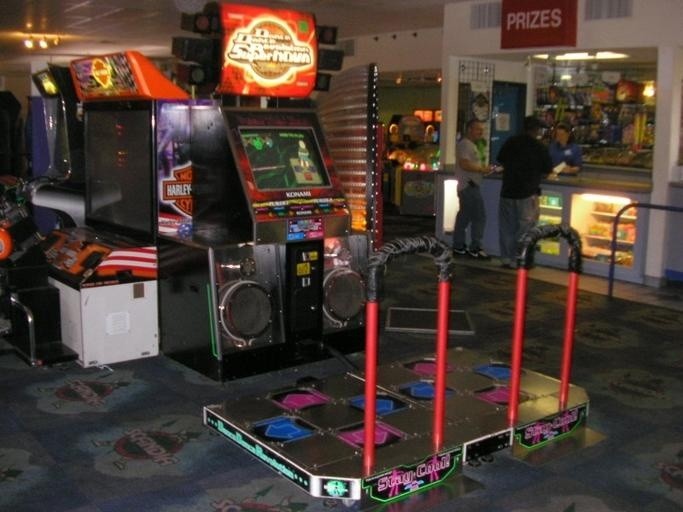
[548,122,581,174]
[453,119,493,260]
[496,116,551,269]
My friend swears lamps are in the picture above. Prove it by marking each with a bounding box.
[39,36,48,50]
[25,36,33,48]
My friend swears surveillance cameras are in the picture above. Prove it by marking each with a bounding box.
[53,37,61,46]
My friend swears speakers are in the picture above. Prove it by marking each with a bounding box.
[220,280,274,341]
[323,269,366,321]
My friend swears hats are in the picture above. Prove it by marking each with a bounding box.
[555,122,573,132]
[524,116,548,129]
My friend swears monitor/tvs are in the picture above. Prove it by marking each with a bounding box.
[81,101,156,244]
[236,125,333,192]
[42,97,72,174]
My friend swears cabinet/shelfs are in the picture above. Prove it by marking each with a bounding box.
[533,178,653,284]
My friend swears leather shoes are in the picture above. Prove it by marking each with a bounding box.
[452,249,470,258]
[470,249,490,261]
